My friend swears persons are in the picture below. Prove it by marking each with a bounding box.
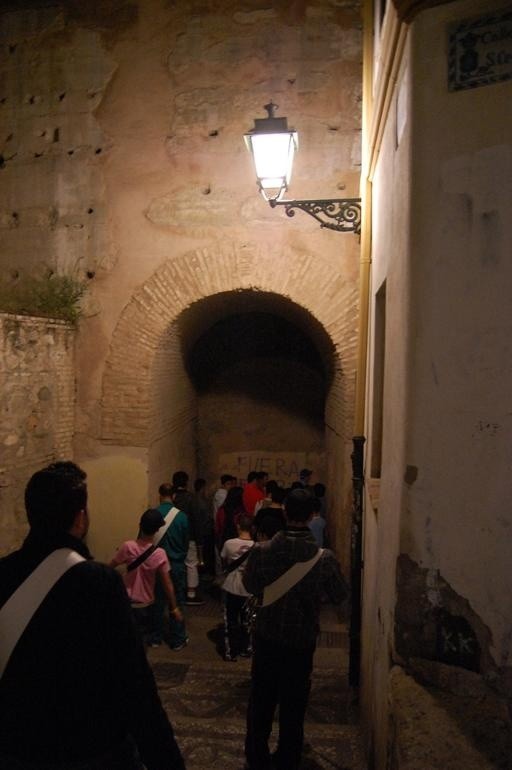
[236,487,351,770]
[0,461,193,769]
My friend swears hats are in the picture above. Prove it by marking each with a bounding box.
[300,469,312,480]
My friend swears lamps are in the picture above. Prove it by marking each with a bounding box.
[240,98,364,238]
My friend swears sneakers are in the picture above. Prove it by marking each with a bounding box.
[184,595,208,607]
[149,635,192,655]
